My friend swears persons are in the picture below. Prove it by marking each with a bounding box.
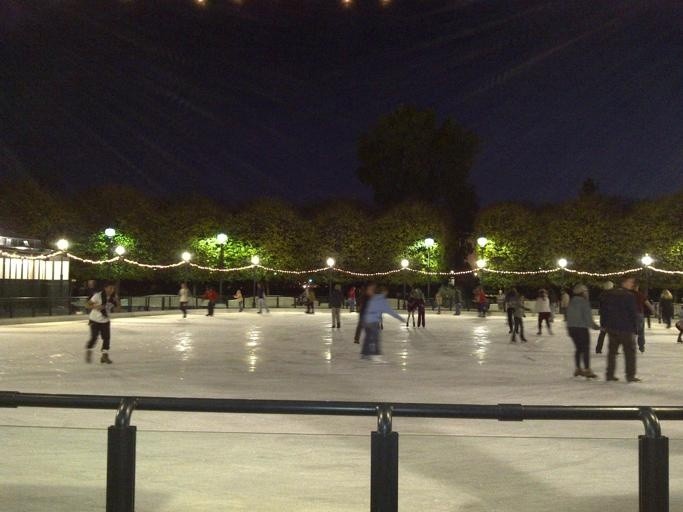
[565,280,681,383]
[176,282,269,317]
[85,283,120,364]
[453,285,570,342]
[305,279,427,359]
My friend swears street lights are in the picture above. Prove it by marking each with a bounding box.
[182,250,195,281]
[400,257,409,309]
[101,226,125,279]
[639,252,652,300]
[114,245,128,296]
[216,232,229,290]
[475,237,487,291]
[252,257,261,296]
[422,238,435,297]
[557,256,570,285]
[55,237,69,303]
[326,256,335,308]
